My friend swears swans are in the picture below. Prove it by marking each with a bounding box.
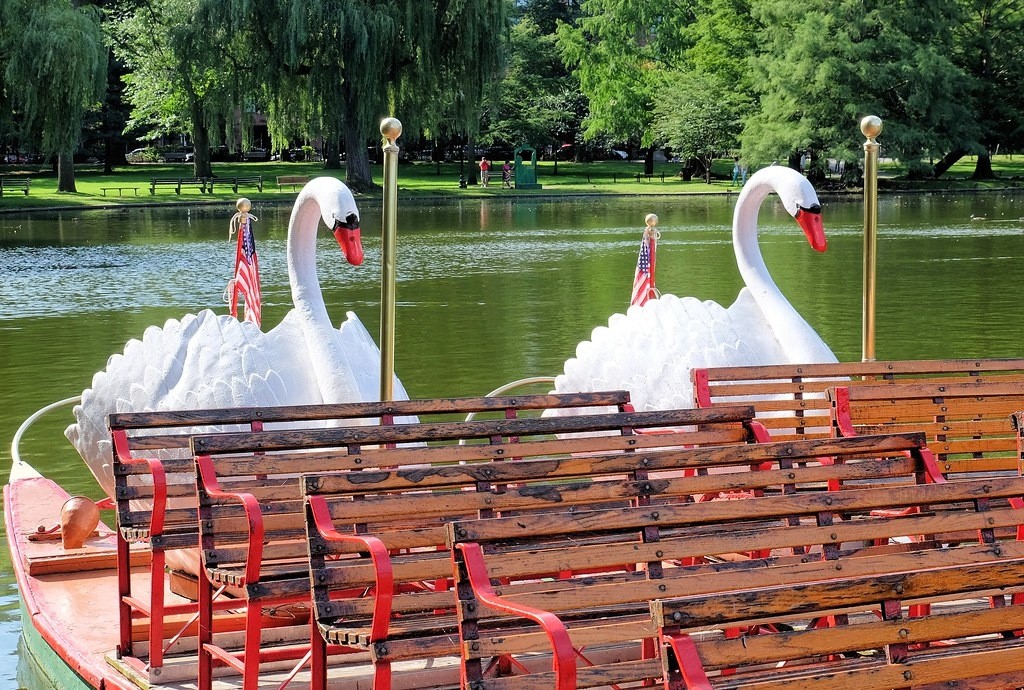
[63,176,432,578]
[540,166,851,502]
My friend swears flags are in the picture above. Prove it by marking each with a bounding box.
[223,212,262,327]
[630,228,654,308]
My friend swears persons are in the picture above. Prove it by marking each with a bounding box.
[740,158,748,186]
[502,160,515,189]
[458,175,467,188]
[731,157,740,187]
[479,157,490,187]
[800,152,807,173]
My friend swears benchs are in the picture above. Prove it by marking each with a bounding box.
[298,432,1020,689]
[647,558,1024,688]
[244,152,266,161]
[442,476,1024,689]
[148,176,208,196]
[276,176,308,192]
[633,171,669,183]
[824,381,1023,548]
[207,175,262,193]
[100,188,140,196]
[190,405,776,690]
[690,359,1024,559]
[0,177,31,195]
[487,170,515,182]
[105,391,688,686]
[991,170,1004,176]
[653,151,668,160]
[729,168,751,179]
[164,152,187,162]
